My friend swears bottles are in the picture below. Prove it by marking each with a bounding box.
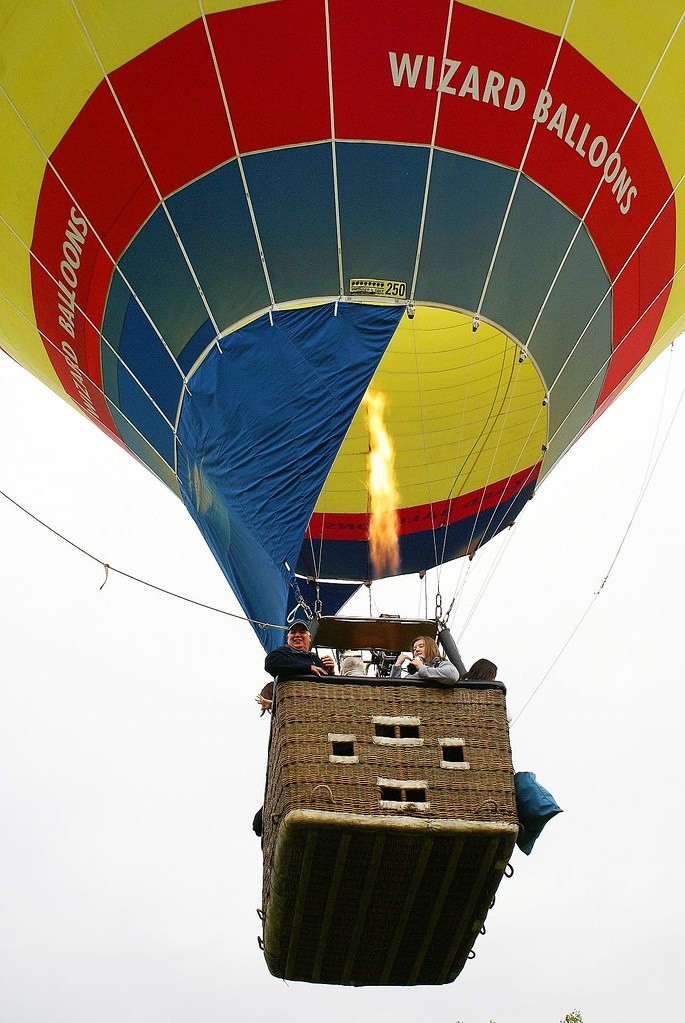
[407,656,424,675]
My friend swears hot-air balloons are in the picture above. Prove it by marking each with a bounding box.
[0,1,685,987]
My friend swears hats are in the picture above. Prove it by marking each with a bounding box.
[288,619,311,633]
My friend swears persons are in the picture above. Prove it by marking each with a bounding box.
[255,682,274,717]
[265,619,335,678]
[390,636,460,685]
